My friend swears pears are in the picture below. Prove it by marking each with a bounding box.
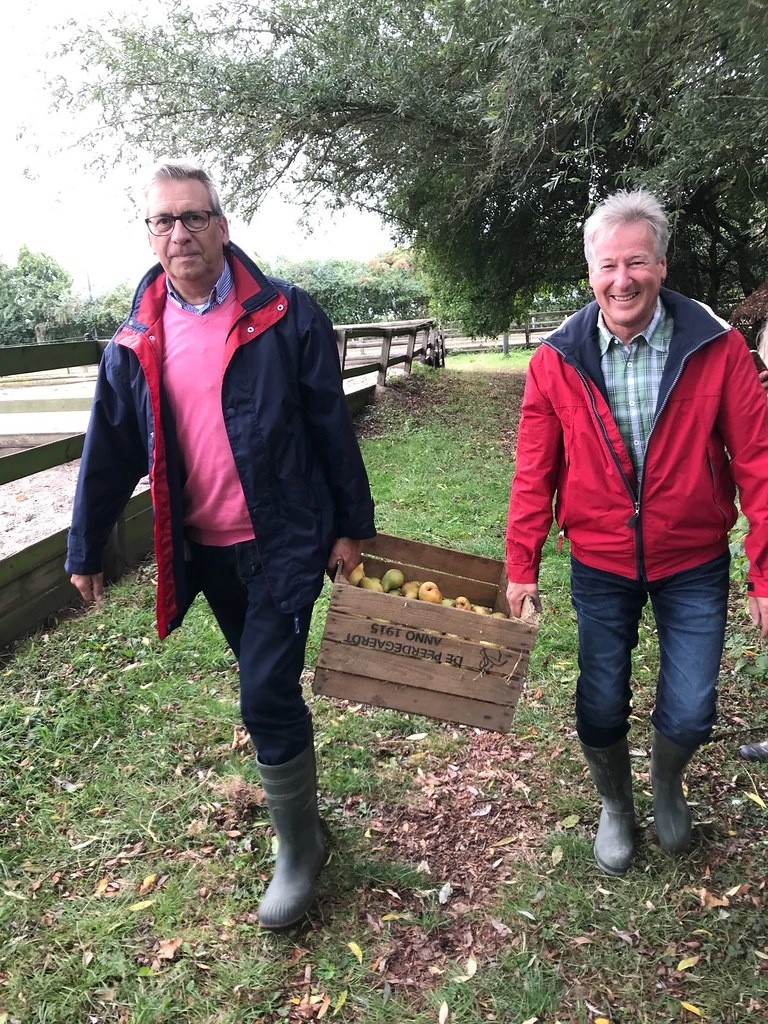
[347,564,510,648]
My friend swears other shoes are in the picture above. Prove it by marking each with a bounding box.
[739,737,768,761]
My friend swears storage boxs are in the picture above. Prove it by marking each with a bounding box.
[310,531,533,734]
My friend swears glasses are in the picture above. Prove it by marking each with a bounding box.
[143,208,217,238]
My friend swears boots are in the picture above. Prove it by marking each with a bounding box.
[646,720,702,850]
[253,703,328,930]
[574,730,636,875]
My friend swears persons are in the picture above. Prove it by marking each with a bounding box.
[66,164,376,928]
[506,191,768,877]
